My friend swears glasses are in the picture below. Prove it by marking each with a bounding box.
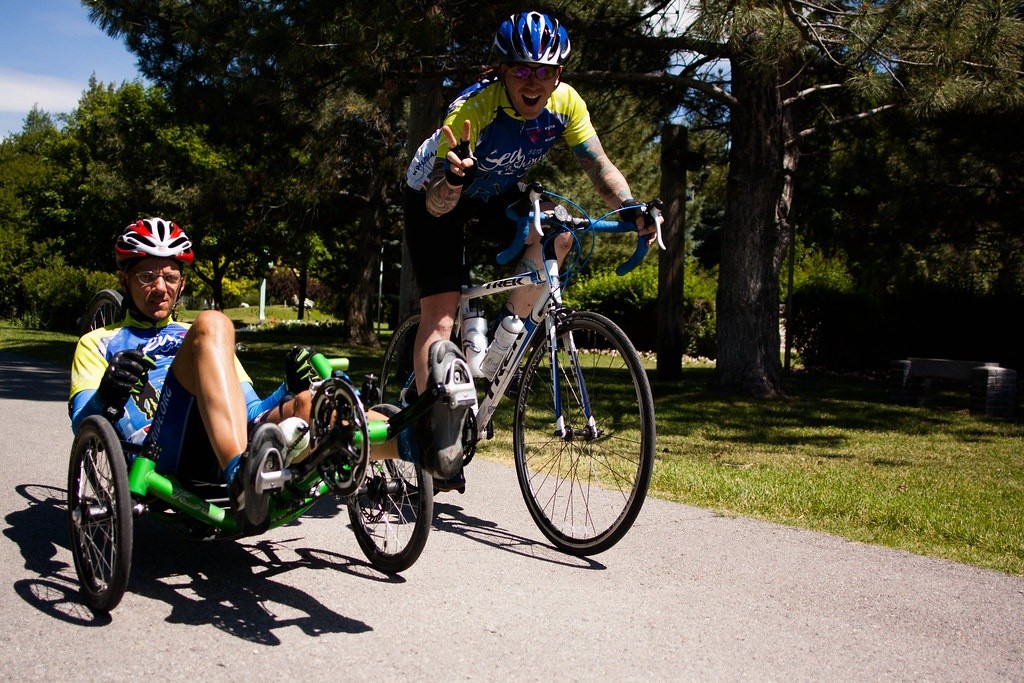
[127,271,184,286]
[504,65,561,81]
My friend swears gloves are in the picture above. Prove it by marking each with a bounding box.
[95,350,158,410]
[286,344,320,392]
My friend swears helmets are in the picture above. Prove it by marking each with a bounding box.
[115,218,194,268]
[493,11,571,67]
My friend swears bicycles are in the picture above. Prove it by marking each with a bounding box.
[379,153,667,559]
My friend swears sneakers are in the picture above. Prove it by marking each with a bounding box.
[410,338,469,479]
[232,422,287,533]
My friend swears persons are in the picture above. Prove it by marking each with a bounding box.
[404,11,665,489]
[69,218,469,521]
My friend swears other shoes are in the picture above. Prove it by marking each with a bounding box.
[432,473,465,492]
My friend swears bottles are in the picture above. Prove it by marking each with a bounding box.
[278,416,310,469]
[462,306,488,377]
[480,314,524,379]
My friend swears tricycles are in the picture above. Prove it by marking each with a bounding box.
[67,290,433,615]
